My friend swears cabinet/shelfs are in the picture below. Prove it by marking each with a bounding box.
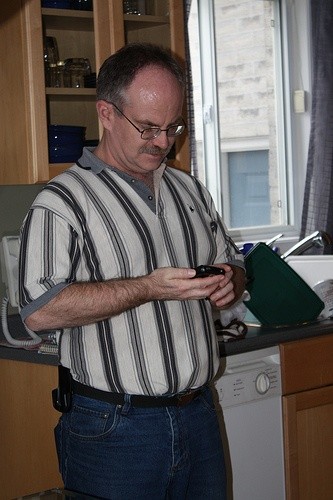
[0,0,191,185]
[283,387,333,500]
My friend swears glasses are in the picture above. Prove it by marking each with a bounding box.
[106,101,186,140]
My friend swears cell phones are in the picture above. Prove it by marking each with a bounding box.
[190,265,225,279]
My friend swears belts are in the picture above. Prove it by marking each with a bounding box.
[71,380,209,408]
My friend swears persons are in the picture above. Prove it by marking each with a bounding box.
[19,42,247,500]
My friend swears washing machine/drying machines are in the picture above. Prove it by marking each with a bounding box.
[214,345,285,500]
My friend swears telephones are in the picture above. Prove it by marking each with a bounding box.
[0,235,19,307]
